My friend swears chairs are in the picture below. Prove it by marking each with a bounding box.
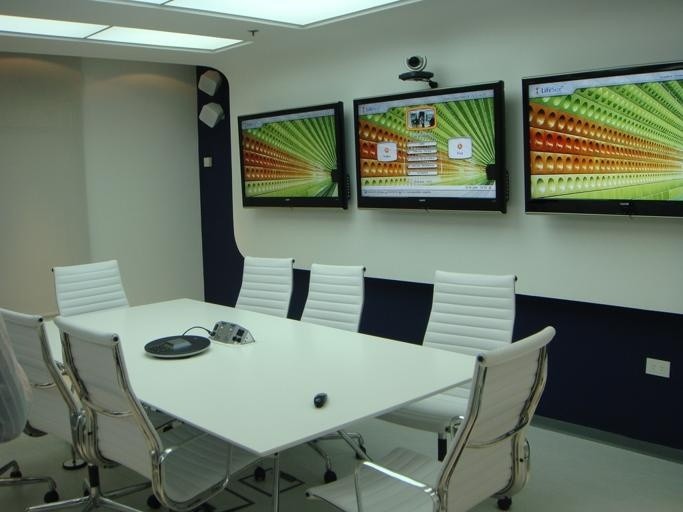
[53,315,264,512]
[306,324,556,512]
[233,257,294,317]
[0,308,149,512]
[299,264,365,480]
[50,261,131,316]
[377,270,518,466]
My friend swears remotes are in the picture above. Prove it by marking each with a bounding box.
[313,392,328,409]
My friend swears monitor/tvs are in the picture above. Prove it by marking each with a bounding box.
[353,81,508,214]
[237,102,350,209]
[521,61,682,217]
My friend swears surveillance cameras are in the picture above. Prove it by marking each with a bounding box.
[404,54,428,71]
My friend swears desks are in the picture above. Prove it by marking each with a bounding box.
[35,297,488,461]
